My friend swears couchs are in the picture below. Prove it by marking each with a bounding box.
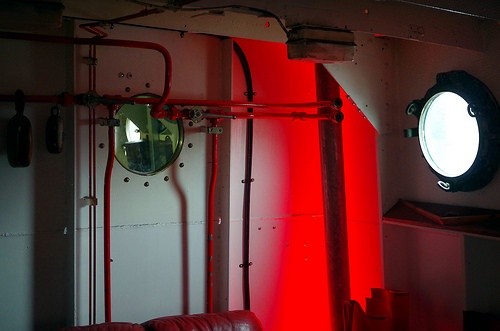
[62,309,264,331]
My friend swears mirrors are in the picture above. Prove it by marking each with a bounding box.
[113,93,185,176]
